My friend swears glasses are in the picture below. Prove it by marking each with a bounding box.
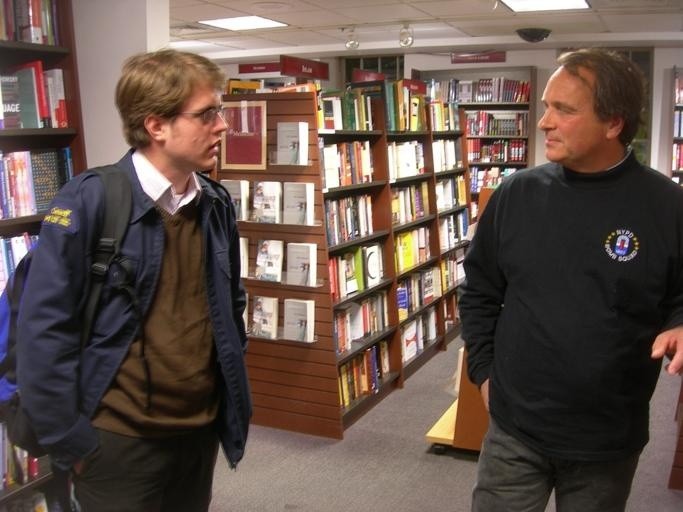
[166,101,226,126]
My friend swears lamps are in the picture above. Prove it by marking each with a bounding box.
[344,27,359,50]
[399,21,415,48]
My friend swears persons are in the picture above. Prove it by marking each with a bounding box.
[14,48,253,512]
[456,48,682,512]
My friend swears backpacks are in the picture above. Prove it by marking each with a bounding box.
[0,165,134,455]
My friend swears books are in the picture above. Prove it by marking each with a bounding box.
[196,73,316,343]
[315,77,530,412]
[668,75,683,189]
[0,0,82,512]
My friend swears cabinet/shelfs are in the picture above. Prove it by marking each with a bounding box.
[202,68,471,442]
[0,0,88,512]
[669,64,683,189]
[410,65,535,221]
[422,347,489,456]
